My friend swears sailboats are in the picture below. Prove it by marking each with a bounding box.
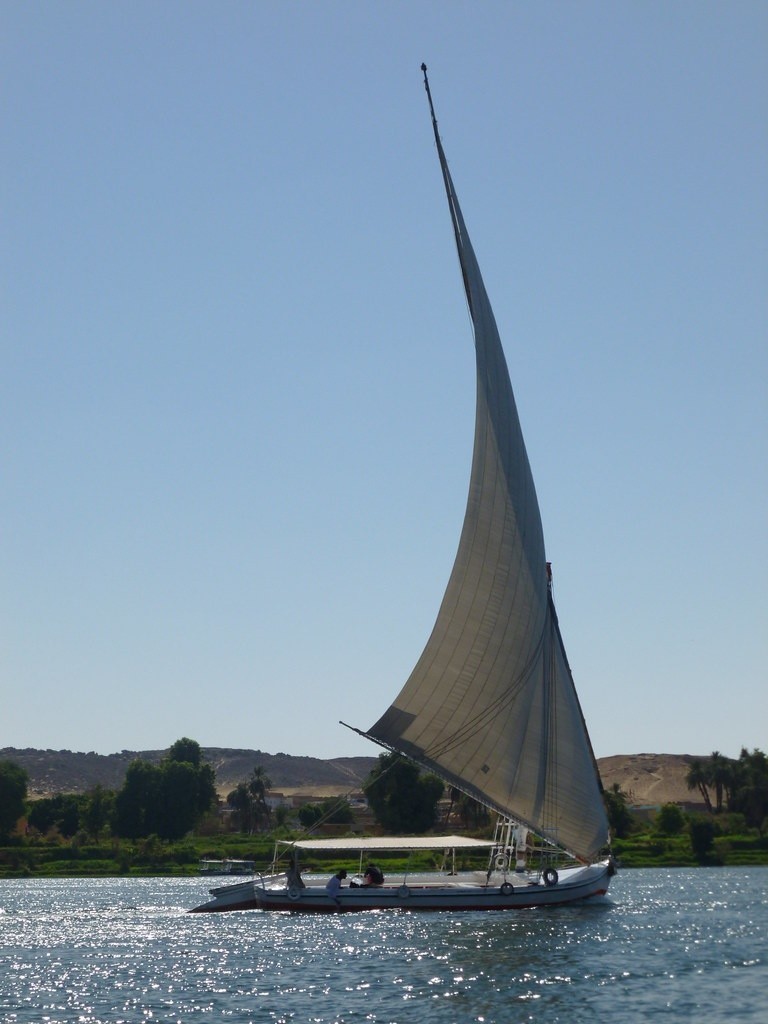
[184,62,618,913]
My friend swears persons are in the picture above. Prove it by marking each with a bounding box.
[364,864,384,884]
[286,861,304,888]
[326,870,346,889]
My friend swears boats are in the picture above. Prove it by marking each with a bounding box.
[198,860,256,876]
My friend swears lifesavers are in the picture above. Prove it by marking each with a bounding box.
[397,886,411,898]
[500,882,515,897]
[493,852,510,870]
[543,868,559,886]
[287,887,302,901]
